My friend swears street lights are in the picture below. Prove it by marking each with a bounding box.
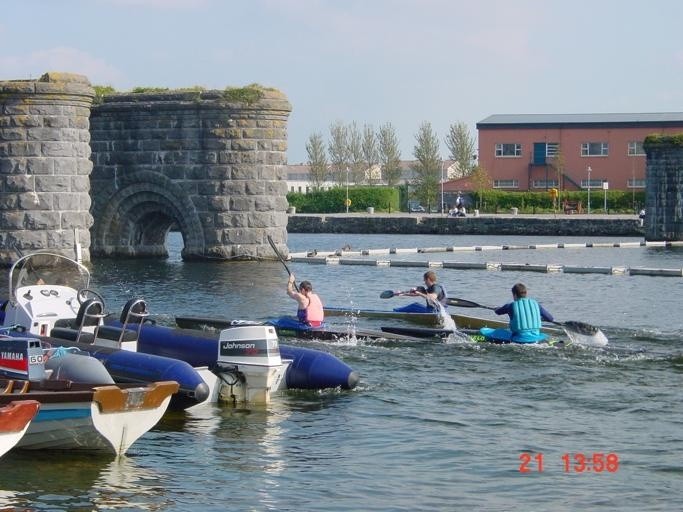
[439,155,445,214]
[585,166,592,213]
[345,166,349,213]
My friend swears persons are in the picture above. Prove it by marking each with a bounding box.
[273,273,324,328]
[393,270,446,313]
[494,283,546,345]
[454,191,465,218]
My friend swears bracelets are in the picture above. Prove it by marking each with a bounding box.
[287,281,292,284]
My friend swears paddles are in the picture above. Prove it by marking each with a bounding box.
[268,235,298,295]
[379,287,424,300]
[444,297,598,336]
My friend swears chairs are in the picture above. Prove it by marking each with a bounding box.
[94,298,150,353]
[50,299,108,344]
